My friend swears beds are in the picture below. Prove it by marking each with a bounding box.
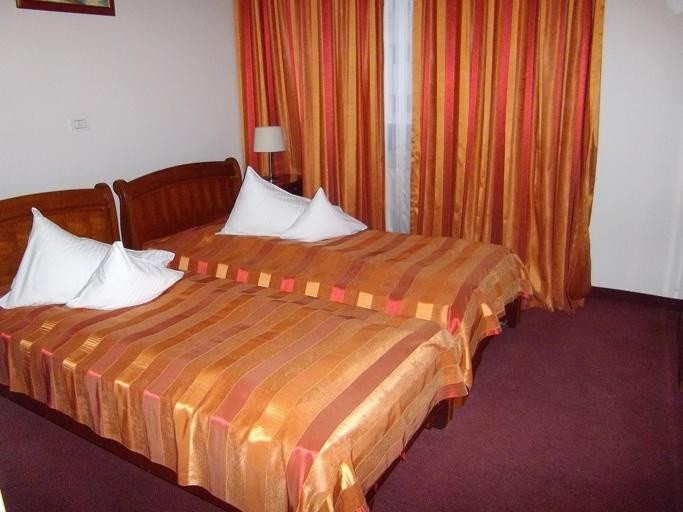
[0,182,467,511]
[111,158,534,376]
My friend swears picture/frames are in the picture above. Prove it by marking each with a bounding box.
[12,0,117,16]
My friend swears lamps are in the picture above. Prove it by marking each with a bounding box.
[250,126,288,182]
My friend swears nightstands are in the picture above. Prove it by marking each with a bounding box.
[262,176,302,197]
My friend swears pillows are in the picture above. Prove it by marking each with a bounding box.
[65,238,184,311]
[279,186,369,244]
[213,166,310,239]
[0,207,175,310]
